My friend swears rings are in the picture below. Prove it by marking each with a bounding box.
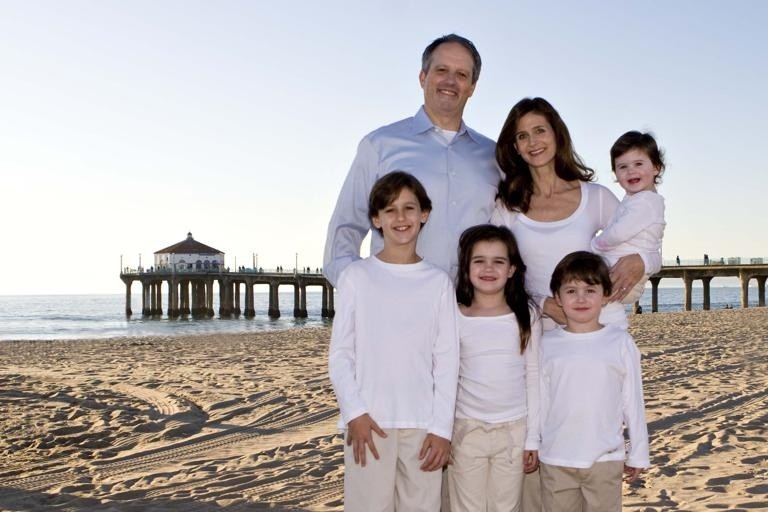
[620,287,625,292]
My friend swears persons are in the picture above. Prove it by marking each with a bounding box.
[330,172,459,512]
[490,98,661,334]
[539,252,650,512]
[592,131,666,304]
[448,225,543,512]
[323,33,506,287]
